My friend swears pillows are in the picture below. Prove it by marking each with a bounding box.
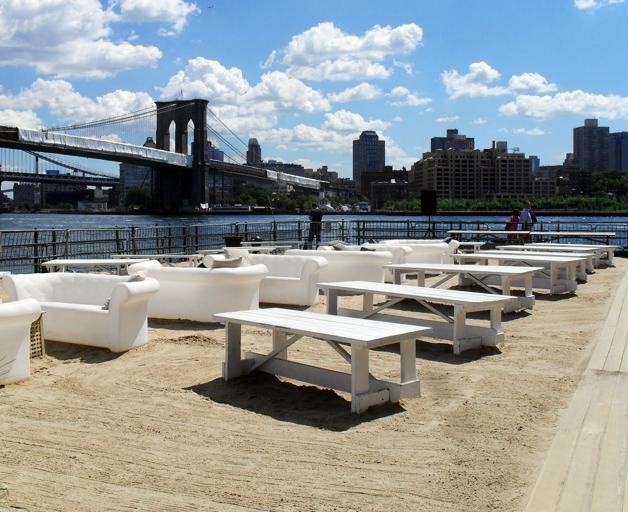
[361,247,377,251]
[269,247,287,254]
[333,244,348,251]
[213,256,243,269]
[248,249,269,254]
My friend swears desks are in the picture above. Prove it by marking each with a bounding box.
[110,254,204,268]
[195,248,277,254]
[31,310,47,359]
[449,253,587,296]
[496,246,602,267]
[42,258,150,275]
[381,262,545,313]
[223,245,292,253]
[479,249,597,281]
[213,308,433,414]
[315,280,519,354]
[447,230,616,245]
[524,243,619,267]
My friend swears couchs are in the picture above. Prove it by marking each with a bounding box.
[284,250,392,283]
[126,259,268,326]
[382,239,460,264]
[1,273,161,354]
[203,251,329,306]
[2,298,41,384]
[317,245,412,282]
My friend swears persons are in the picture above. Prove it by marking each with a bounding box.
[517,200,533,244]
[307,203,324,249]
[506,209,521,239]
